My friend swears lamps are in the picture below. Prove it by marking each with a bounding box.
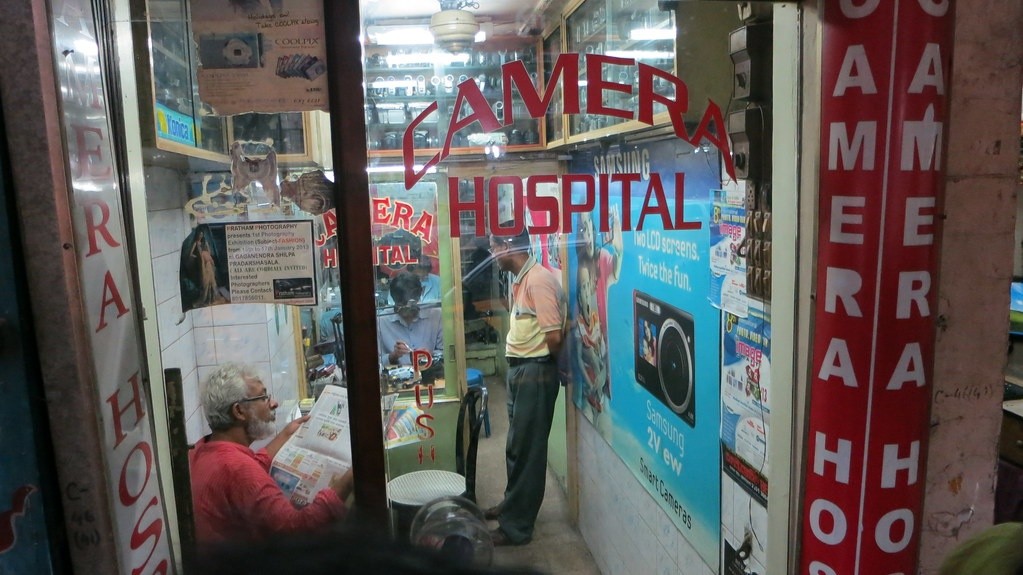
[430,0,480,54]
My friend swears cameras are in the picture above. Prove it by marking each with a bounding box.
[632,288,696,429]
[200,32,263,70]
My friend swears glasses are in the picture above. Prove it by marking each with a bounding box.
[487,243,499,254]
[238,390,271,402]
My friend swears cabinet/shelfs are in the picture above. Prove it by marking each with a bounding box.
[362,0,676,157]
[129,0,334,170]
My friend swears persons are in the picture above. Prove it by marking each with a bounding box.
[378,272,444,374]
[190,362,353,549]
[189,230,219,306]
[573,203,622,441]
[388,255,440,304]
[483,220,566,545]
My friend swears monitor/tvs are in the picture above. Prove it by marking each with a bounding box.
[1009,275,1023,344]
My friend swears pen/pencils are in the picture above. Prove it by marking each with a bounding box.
[517,310,519,315]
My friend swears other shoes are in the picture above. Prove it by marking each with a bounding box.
[489,528,532,546]
[481,507,500,519]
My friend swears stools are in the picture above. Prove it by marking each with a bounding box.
[466,368,490,438]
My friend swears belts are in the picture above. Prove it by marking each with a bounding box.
[506,355,549,366]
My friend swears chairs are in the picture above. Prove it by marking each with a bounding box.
[386,385,489,544]
